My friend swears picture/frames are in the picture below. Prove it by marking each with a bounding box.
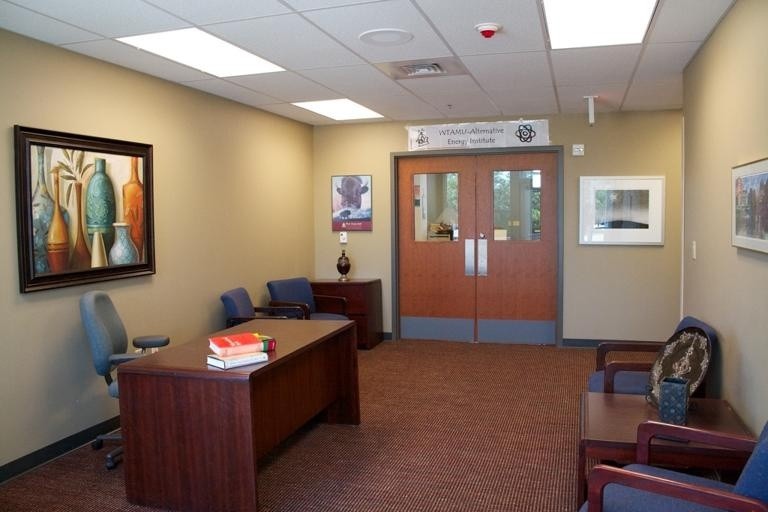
[12,124,156,295]
[578,174,667,248]
[331,174,374,233]
[730,158,768,253]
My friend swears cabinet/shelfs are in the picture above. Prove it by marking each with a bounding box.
[309,277,384,350]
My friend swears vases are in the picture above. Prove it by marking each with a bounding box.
[33,144,146,273]
[336,247,353,280]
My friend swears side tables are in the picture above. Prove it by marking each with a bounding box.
[575,390,755,510]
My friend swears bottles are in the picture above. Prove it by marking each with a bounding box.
[337,250,351,281]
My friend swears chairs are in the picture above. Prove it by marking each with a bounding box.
[267,276,348,321]
[576,418,764,510]
[79,290,170,470]
[219,287,304,325]
[586,316,719,393]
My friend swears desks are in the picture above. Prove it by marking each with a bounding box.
[115,316,362,512]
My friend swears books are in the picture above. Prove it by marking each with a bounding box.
[209,332,275,357]
[207,351,269,371]
